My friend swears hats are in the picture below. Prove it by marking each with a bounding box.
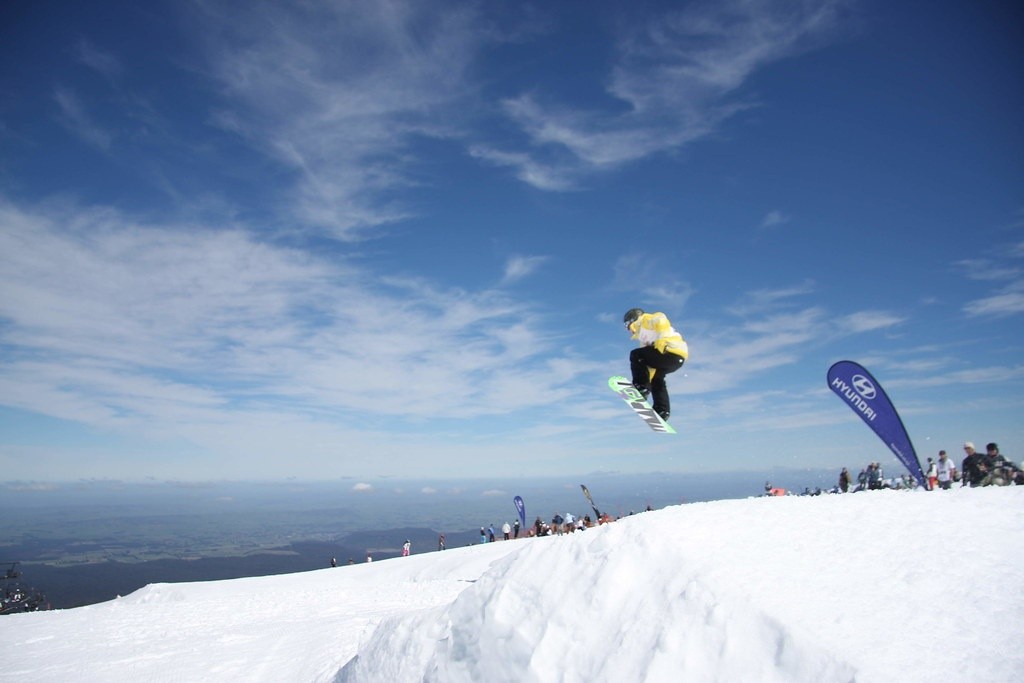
[624,309,643,330]
[964,442,974,449]
[987,443,999,453]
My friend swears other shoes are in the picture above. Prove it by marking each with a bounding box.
[634,384,650,394]
[657,411,669,420]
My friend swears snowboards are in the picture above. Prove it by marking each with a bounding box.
[607,375,678,434]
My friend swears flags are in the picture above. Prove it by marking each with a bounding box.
[513,496,526,526]
[580,484,602,519]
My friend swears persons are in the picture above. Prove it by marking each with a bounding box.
[480,519,520,544]
[924,441,1024,491]
[438,532,446,552]
[401,539,411,557]
[330,554,372,567]
[622,309,689,422]
[527,510,592,537]
[0,585,50,613]
[596,505,653,524]
[765,461,919,498]
